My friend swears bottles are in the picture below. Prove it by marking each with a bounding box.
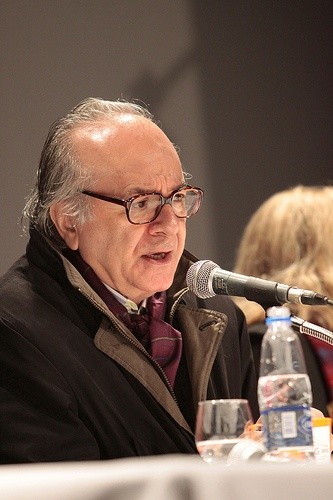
[257,305,317,462]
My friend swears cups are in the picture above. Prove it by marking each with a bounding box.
[195,399,255,462]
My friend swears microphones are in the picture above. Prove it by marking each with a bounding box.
[186,260,329,306]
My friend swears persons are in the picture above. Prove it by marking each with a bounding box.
[0,97,260,464]
[226,183,332,436]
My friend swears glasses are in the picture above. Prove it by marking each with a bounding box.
[78,181,204,225]
